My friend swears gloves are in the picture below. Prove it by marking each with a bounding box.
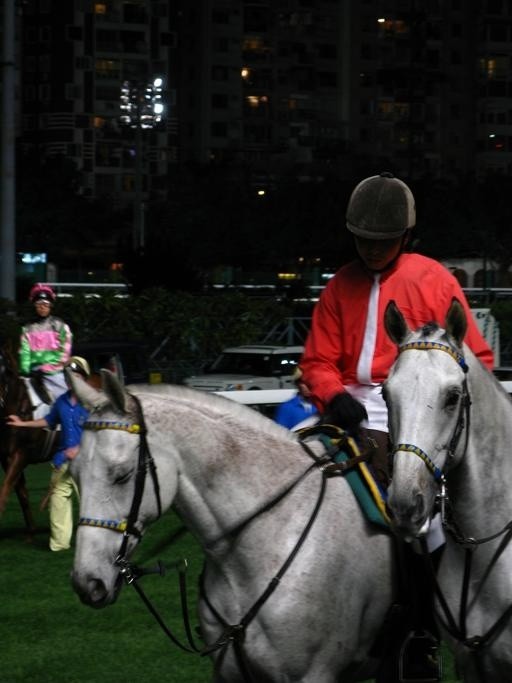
[327,392,368,432]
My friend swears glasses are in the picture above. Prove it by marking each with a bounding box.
[34,299,52,307]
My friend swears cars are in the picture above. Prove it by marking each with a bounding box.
[184,344,306,392]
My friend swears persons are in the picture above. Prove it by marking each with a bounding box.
[15,282,75,404]
[4,354,96,553]
[272,169,495,681]
[273,363,323,431]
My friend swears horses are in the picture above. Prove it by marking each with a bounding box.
[60,366,442,682]
[369,296,512,683]
[0,356,125,544]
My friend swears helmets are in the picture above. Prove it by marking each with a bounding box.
[28,282,56,301]
[64,355,91,377]
[345,171,416,239]
[293,366,303,380]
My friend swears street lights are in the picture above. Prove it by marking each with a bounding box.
[118,71,165,257]
